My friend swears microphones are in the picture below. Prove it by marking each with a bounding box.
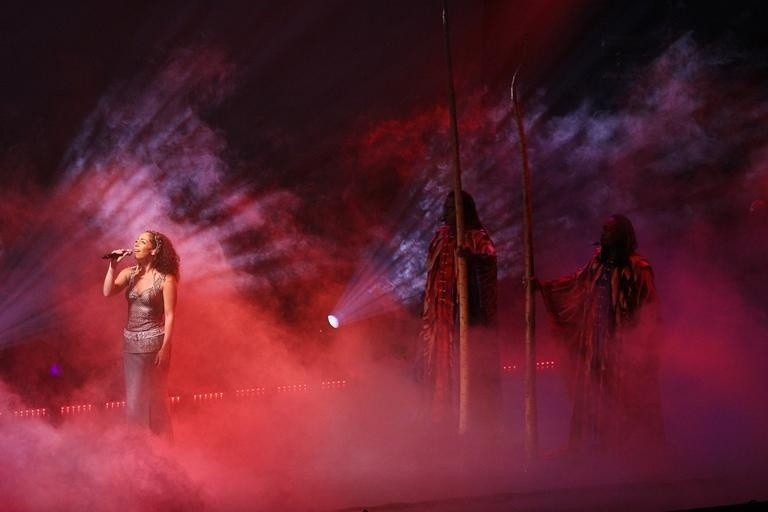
[102,248,133,259]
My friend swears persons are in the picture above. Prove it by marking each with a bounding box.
[102,230,181,437]
[521,214,665,444]
[417,187,504,436]
